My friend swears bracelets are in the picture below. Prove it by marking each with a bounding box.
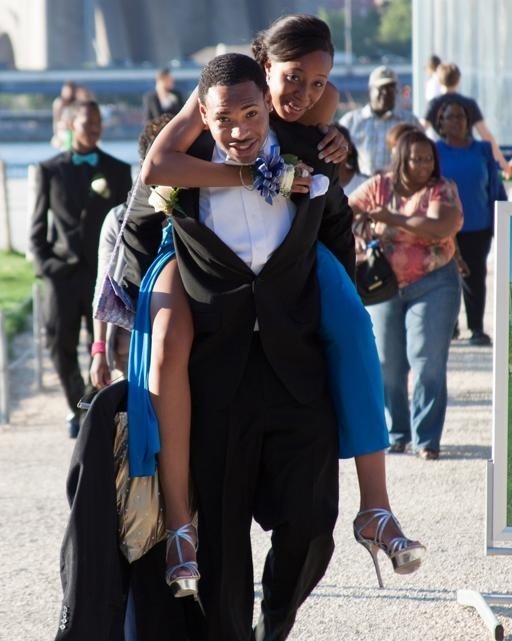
[238,164,248,188]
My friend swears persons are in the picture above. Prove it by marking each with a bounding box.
[24,70,183,439]
[117,54,357,641]
[340,58,511,463]
[142,13,426,597]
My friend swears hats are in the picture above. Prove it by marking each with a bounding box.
[368,66,398,88]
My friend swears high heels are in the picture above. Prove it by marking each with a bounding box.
[352,508,427,589]
[163,522,201,598]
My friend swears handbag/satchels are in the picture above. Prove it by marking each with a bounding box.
[355,253,399,306]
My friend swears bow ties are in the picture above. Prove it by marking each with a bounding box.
[71,153,99,168]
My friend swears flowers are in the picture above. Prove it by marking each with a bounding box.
[252,144,297,205]
[146,185,179,219]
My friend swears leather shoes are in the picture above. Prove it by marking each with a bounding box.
[386,444,439,459]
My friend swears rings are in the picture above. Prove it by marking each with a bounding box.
[301,169,308,178]
[342,143,350,150]
